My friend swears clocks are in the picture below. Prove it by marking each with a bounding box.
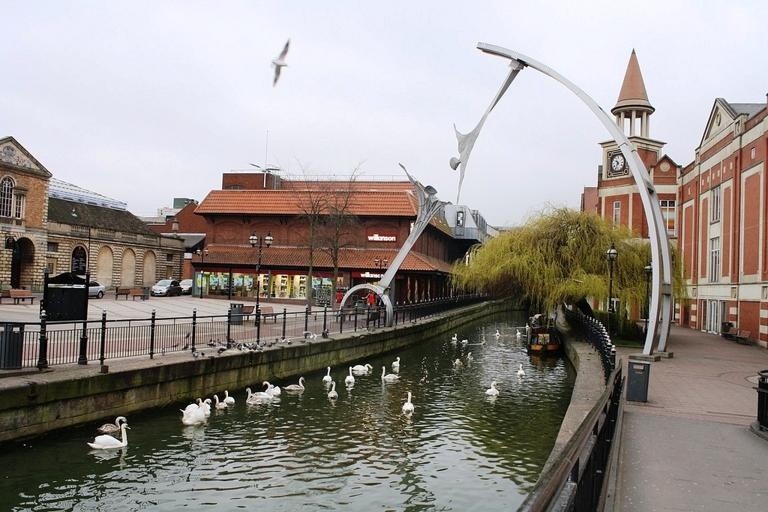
[611,155,625,172]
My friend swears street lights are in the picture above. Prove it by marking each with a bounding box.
[643,263,653,346]
[68,208,91,288]
[606,242,617,338]
[196,248,209,297]
[249,232,274,325]
[248,162,280,189]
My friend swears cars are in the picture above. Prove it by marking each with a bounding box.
[151,279,182,298]
[86,279,105,301]
[179,279,192,294]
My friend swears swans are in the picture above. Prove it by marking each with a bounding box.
[452,334,473,369]
[485,381,499,395]
[402,391,414,418]
[516,328,521,339]
[495,330,500,339]
[517,364,525,376]
[87,423,131,449]
[97,416,127,433]
[322,356,401,400]
[180,376,306,426]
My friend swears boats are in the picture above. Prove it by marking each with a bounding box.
[528,352,562,372]
[525,322,559,354]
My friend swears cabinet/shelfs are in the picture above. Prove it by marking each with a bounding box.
[197,272,332,299]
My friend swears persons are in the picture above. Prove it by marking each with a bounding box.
[336,290,343,310]
[361,289,376,309]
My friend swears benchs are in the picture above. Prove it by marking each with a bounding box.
[733,330,751,344]
[242,306,255,320]
[722,328,738,340]
[115,288,130,300]
[260,307,279,323]
[10,290,35,305]
[130,289,146,301]
[0,290,19,304]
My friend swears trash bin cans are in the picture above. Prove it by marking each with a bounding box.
[626,360,651,403]
[230,303,244,325]
[141,286,150,300]
[0,321,25,370]
[757,369,768,432]
[721,322,729,337]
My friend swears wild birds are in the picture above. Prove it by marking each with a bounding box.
[272,40,289,87]
[171,328,330,358]
